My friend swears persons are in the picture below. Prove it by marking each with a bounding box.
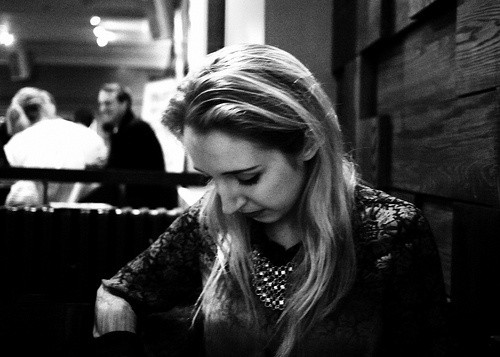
[2,88,107,208]
[87,41,451,357]
[75,82,166,210]
